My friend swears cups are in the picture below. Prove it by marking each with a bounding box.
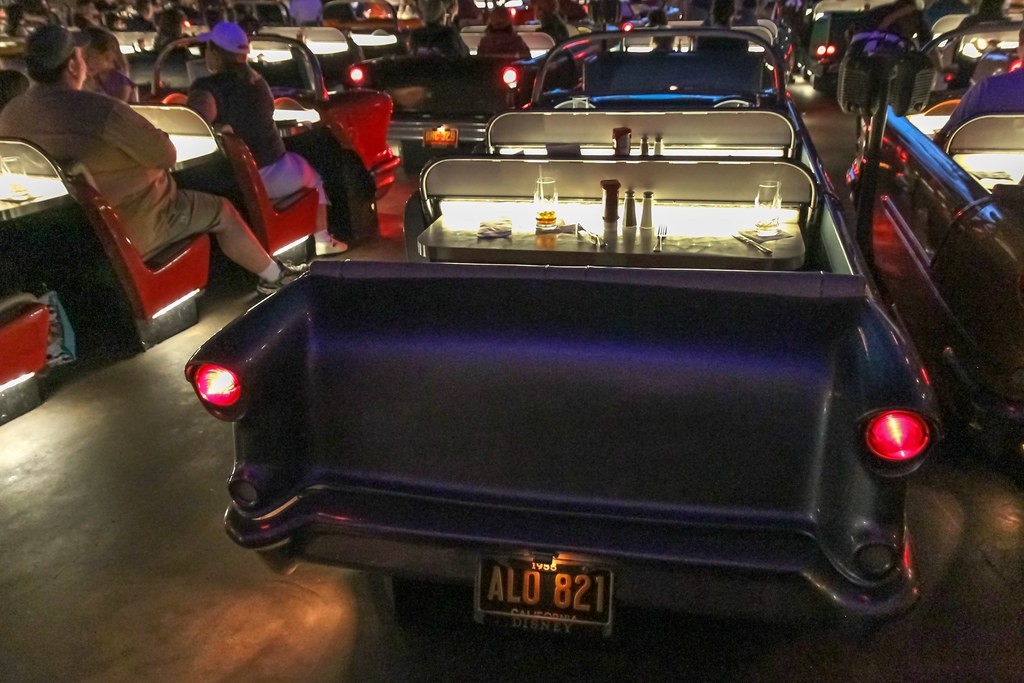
[753,181,783,230]
[601,187,619,221]
[533,177,559,228]
[614,128,631,154]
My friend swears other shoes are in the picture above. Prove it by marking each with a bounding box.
[315,234,349,257]
[258,259,307,294]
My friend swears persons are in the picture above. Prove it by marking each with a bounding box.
[0,24,309,295]
[479,7,532,61]
[846,0,1024,139]
[82,25,137,105]
[534,0,802,82]
[187,25,348,256]
[4,0,471,63]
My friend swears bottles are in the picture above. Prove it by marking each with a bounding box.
[622,190,637,227]
[654,137,662,155]
[640,137,649,155]
[640,192,654,230]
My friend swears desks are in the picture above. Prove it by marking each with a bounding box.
[169,134,221,173]
[273,109,325,137]
[416,145,825,271]
[952,152,1024,187]
[907,113,953,137]
[0,171,71,222]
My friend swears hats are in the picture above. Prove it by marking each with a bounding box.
[26,25,91,69]
[197,23,249,54]
[491,7,513,30]
[422,0,456,24]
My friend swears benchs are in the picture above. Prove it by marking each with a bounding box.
[0,106,319,428]
[150,27,365,104]
[459,24,578,57]
[582,19,778,110]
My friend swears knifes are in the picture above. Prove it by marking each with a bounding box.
[730,231,773,256]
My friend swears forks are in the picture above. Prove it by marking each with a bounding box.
[652,225,668,252]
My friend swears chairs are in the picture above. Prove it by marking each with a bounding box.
[945,112,1024,153]
